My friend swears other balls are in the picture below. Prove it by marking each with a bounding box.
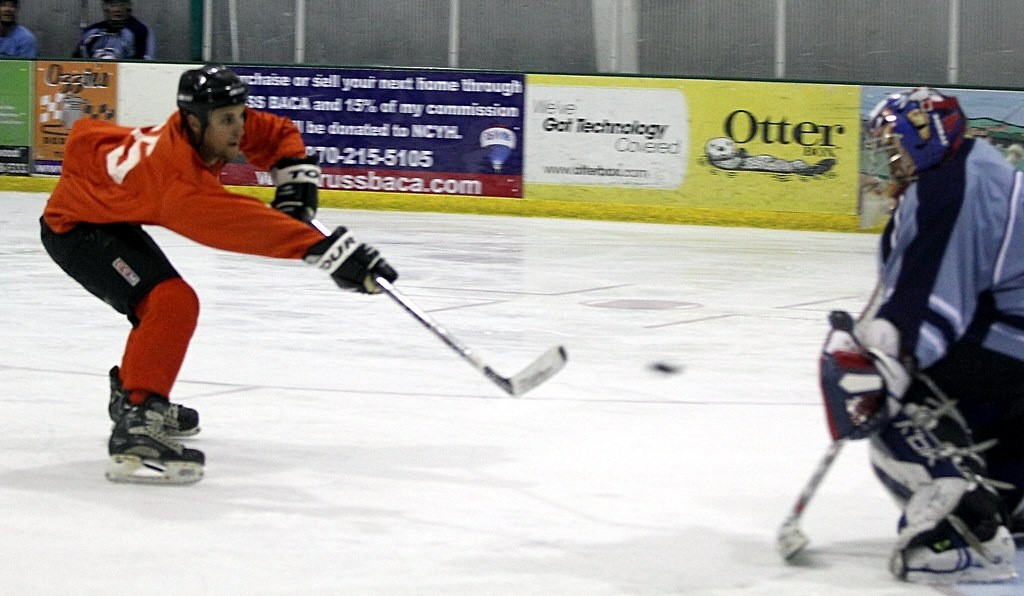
[651,361,678,374]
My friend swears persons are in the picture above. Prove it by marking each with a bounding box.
[0,0,41,61]
[69,0,159,63]
[40,65,400,487]
[814,83,1023,586]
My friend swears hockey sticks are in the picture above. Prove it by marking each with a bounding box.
[775,437,855,563]
[270,198,572,400]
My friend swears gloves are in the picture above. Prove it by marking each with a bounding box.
[303,226,398,294]
[267,151,322,224]
[817,317,916,440]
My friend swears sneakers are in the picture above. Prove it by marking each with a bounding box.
[892,473,1018,585]
[109,365,200,437]
[107,392,206,483]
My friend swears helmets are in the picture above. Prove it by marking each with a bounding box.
[176,62,249,127]
[862,86,969,187]
